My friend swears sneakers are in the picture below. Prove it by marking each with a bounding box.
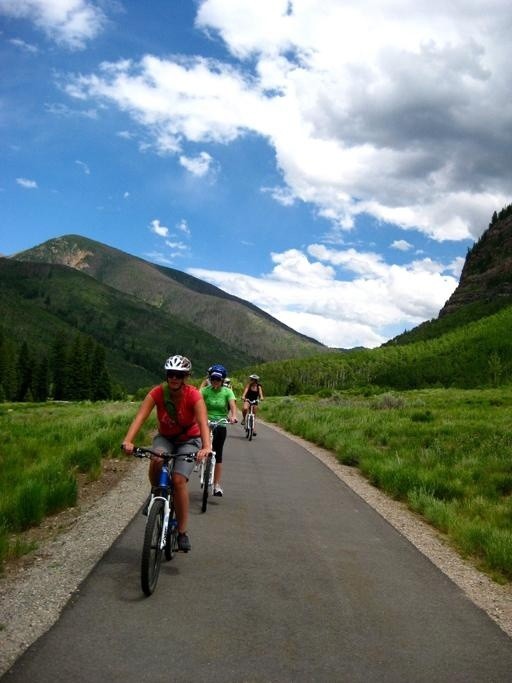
[177,531,191,551]
[241,420,245,425]
[142,492,156,516]
[214,486,223,497]
[253,433,257,436]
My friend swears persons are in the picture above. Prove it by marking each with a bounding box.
[123,354,212,551]
[200,365,236,497]
[241,374,265,435]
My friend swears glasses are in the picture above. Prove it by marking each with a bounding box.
[211,377,222,382]
[167,371,186,379]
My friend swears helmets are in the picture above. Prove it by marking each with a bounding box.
[249,375,259,379]
[164,355,192,371]
[208,365,227,378]
